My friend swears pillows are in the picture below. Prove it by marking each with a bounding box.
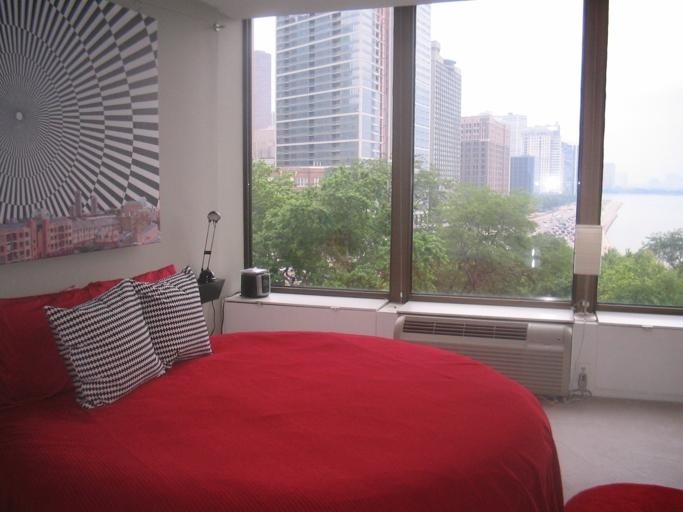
[131,266,213,370]
[43,277,166,410]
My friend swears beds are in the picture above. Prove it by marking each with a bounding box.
[0,264,565,512]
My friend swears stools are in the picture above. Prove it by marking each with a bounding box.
[566,483,683,512]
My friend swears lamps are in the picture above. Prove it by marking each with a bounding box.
[199,211,222,283]
[573,224,602,322]
